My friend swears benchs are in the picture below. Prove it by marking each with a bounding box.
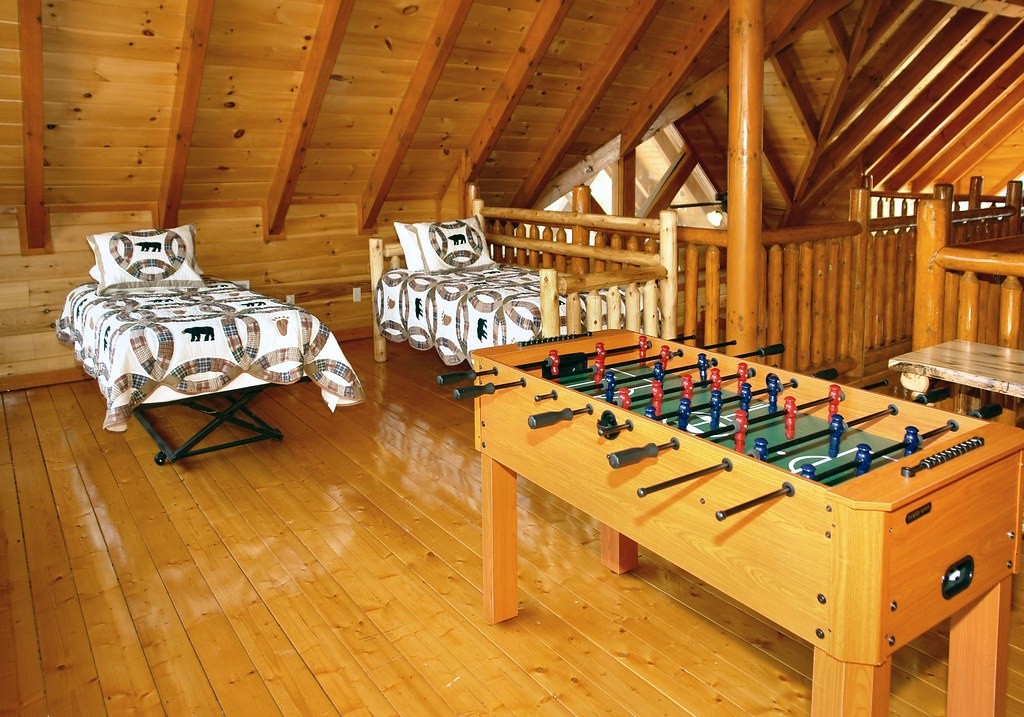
[369,196,679,368]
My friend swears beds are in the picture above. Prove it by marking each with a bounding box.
[56,275,367,465]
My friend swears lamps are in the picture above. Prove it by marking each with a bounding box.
[706,207,724,226]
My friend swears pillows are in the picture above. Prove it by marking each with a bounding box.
[391,215,497,274]
[88,264,204,282]
[85,222,208,296]
